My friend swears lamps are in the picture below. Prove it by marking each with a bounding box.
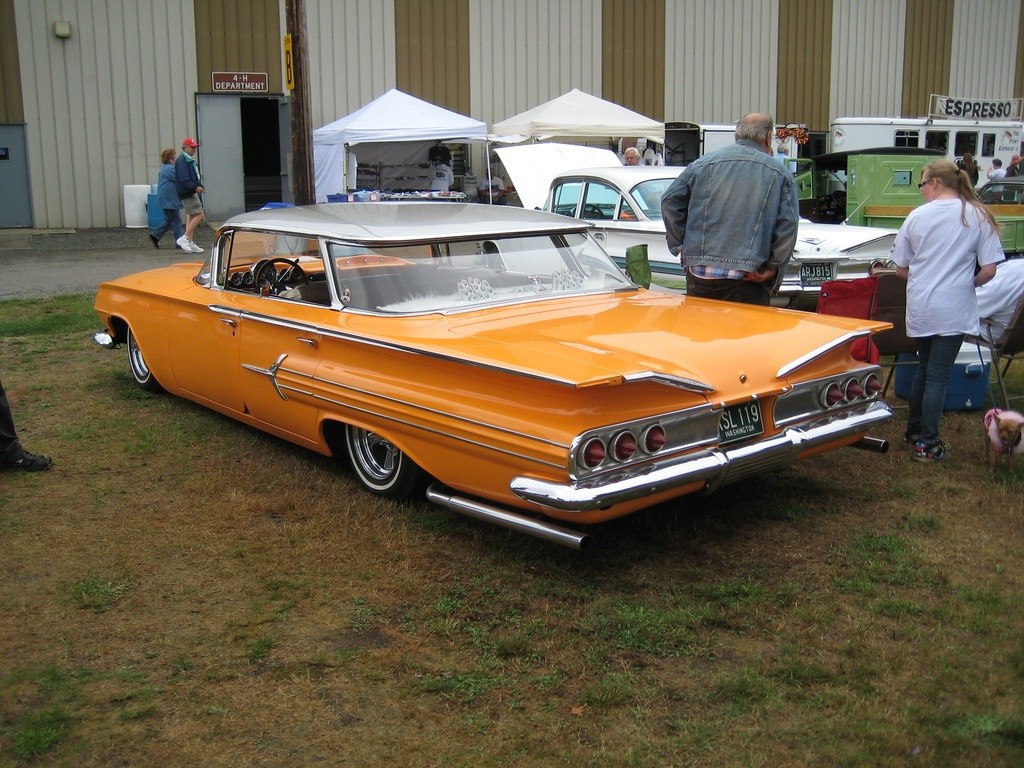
[55,21,71,37]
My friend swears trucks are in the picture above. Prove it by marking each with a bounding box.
[830,116,1024,191]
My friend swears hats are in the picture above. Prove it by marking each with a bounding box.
[1010,154,1024,164]
[182,138,200,147]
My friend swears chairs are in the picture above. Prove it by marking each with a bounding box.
[816,277,889,365]
[441,267,499,296]
[339,273,426,309]
[964,288,1024,411]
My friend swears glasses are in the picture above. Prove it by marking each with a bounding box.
[917,180,939,190]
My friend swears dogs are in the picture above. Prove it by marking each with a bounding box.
[983,409,1024,474]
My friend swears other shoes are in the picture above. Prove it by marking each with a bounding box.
[149,234,160,248]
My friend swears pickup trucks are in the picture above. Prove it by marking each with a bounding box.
[781,146,1024,258]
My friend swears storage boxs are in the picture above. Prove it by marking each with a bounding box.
[893,341,995,412]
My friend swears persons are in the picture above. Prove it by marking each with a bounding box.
[625,147,645,166]
[478,167,507,205]
[0,381,53,471]
[174,138,205,253]
[958,153,978,185]
[888,159,1006,463]
[660,113,799,306]
[775,143,795,173]
[987,159,1007,181]
[428,161,454,201]
[1003,154,1024,200]
[148,149,183,250]
[975,259,1024,345]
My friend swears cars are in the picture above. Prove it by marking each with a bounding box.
[495,140,899,312]
[93,201,897,552]
[976,177,1024,217]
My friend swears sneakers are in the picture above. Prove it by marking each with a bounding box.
[176,237,193,254]
[4,452,51,473]
[190,243,204,253]
[913,440,952,461]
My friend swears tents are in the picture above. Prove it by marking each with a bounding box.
[313,89,492,205]
[491,88,665,160]
